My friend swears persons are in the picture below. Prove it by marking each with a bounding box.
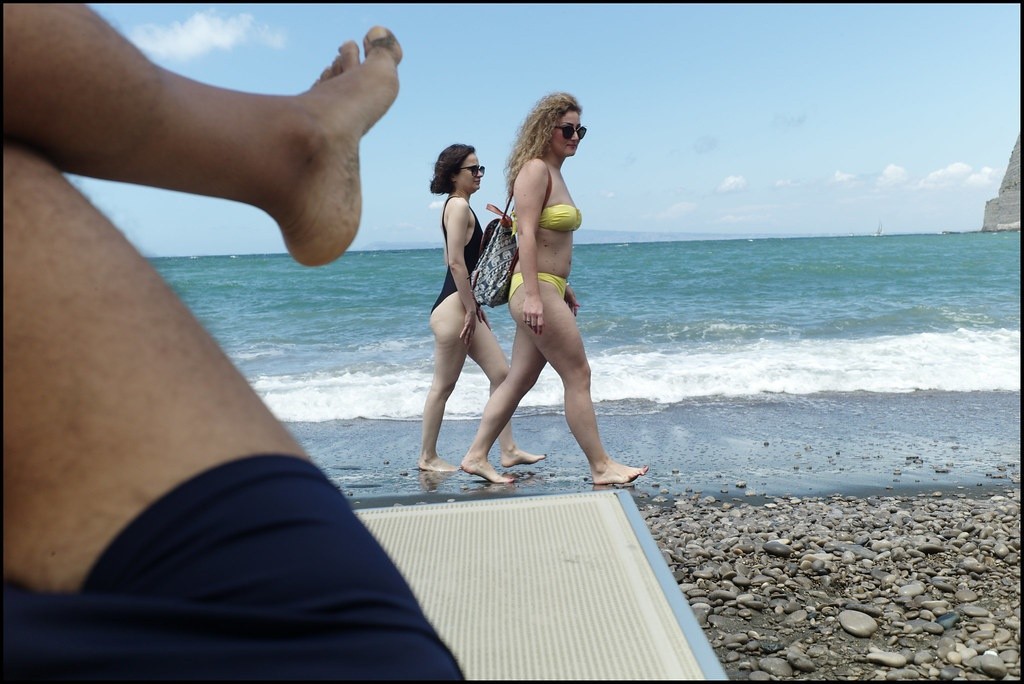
[3,3,467,683]
[461,92,649,484]
[419,142,547,472]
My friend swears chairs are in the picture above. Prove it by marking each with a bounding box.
[350,486,729,683]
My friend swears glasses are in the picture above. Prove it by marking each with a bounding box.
[459,165,485,176]
[557,126,587,139]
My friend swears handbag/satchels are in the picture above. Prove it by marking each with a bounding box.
[470,218,518,306]
